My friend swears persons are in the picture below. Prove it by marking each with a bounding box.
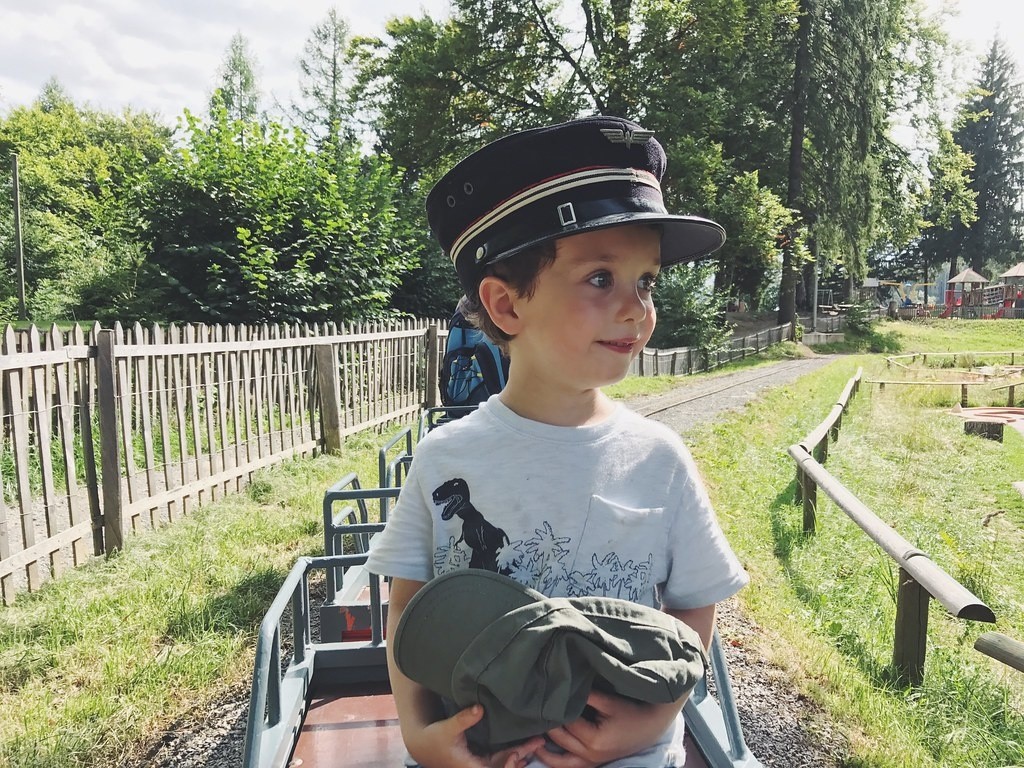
[366,114,744,768]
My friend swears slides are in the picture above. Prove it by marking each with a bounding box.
[982,298,1014,320]
[938,303,953,318]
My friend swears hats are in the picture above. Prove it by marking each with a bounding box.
[425,116,726,297]
[392,569,708,754]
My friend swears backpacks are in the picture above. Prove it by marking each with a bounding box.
[438,304,510,419]
